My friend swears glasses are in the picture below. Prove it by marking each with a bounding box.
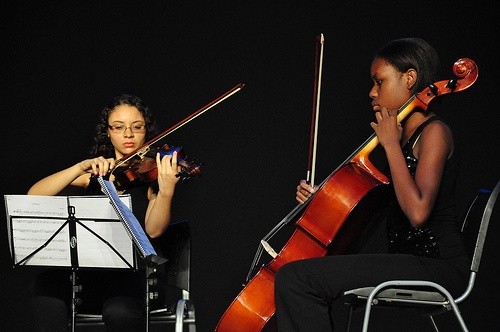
[107,123,149,134]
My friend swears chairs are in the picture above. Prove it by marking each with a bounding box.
[68,220,197,332]
[339,178,500,332]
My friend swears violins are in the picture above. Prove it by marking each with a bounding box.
[111,144,203,189]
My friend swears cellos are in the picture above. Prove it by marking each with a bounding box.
[213,56,480,332]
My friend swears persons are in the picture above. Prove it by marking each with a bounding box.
[26,93,180,332]
[274,37,471,332]
